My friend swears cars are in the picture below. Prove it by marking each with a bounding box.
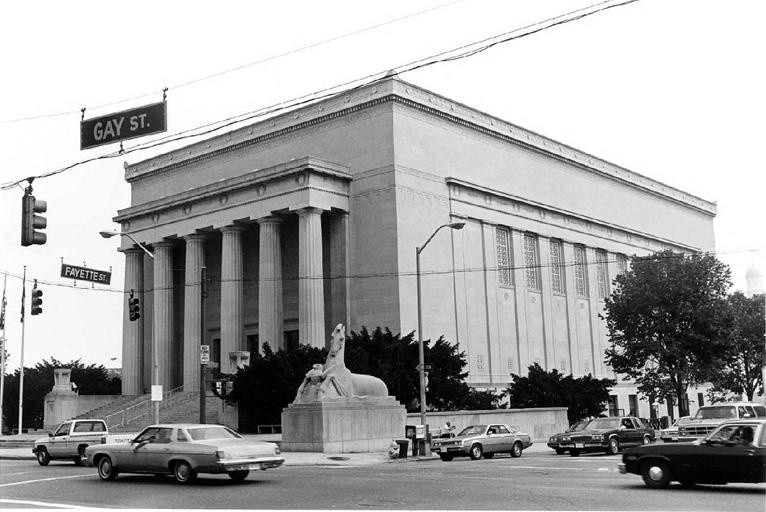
[546,403,766,457]
[81,420,283,485]
[618,420,766,490]
[430,424,533,461]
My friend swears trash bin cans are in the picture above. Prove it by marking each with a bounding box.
[397,440,408,458]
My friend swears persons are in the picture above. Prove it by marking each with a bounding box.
[742,427,754,442]
[438,421,456,438]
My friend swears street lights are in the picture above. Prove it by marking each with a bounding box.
[415,219,466,425]
[100,230,159,428]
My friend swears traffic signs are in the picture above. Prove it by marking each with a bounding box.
[60,264,111,285]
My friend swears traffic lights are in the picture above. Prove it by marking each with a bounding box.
[127,297,139,320]
[30,288,43,315]
[20,195,49,246]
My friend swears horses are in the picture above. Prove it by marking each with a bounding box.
[292,323,389,403]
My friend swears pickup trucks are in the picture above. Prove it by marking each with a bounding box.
[31,418,142,466]
